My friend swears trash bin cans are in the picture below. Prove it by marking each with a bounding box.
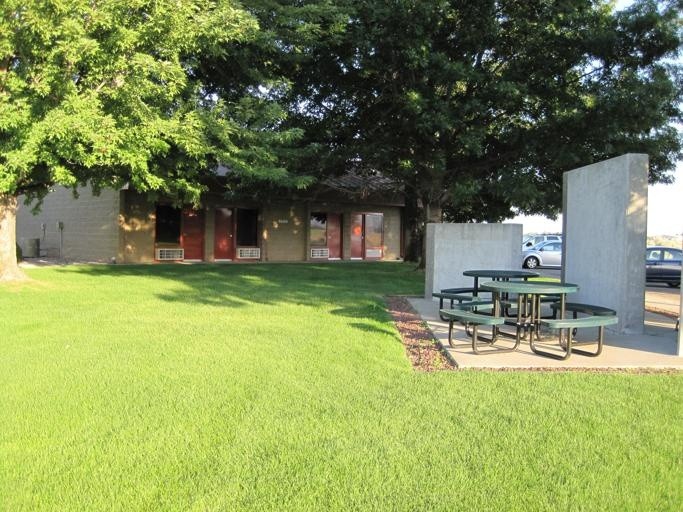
[21,236,40,259]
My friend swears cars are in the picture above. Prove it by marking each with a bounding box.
[519,235,561,270]
[644,247,682,287]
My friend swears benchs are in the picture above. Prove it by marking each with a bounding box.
[430,287,620,359]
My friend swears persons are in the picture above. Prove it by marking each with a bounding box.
[651,251,659,261]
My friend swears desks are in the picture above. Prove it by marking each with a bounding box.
[462,269,539,317]
[479,280,578,344]
